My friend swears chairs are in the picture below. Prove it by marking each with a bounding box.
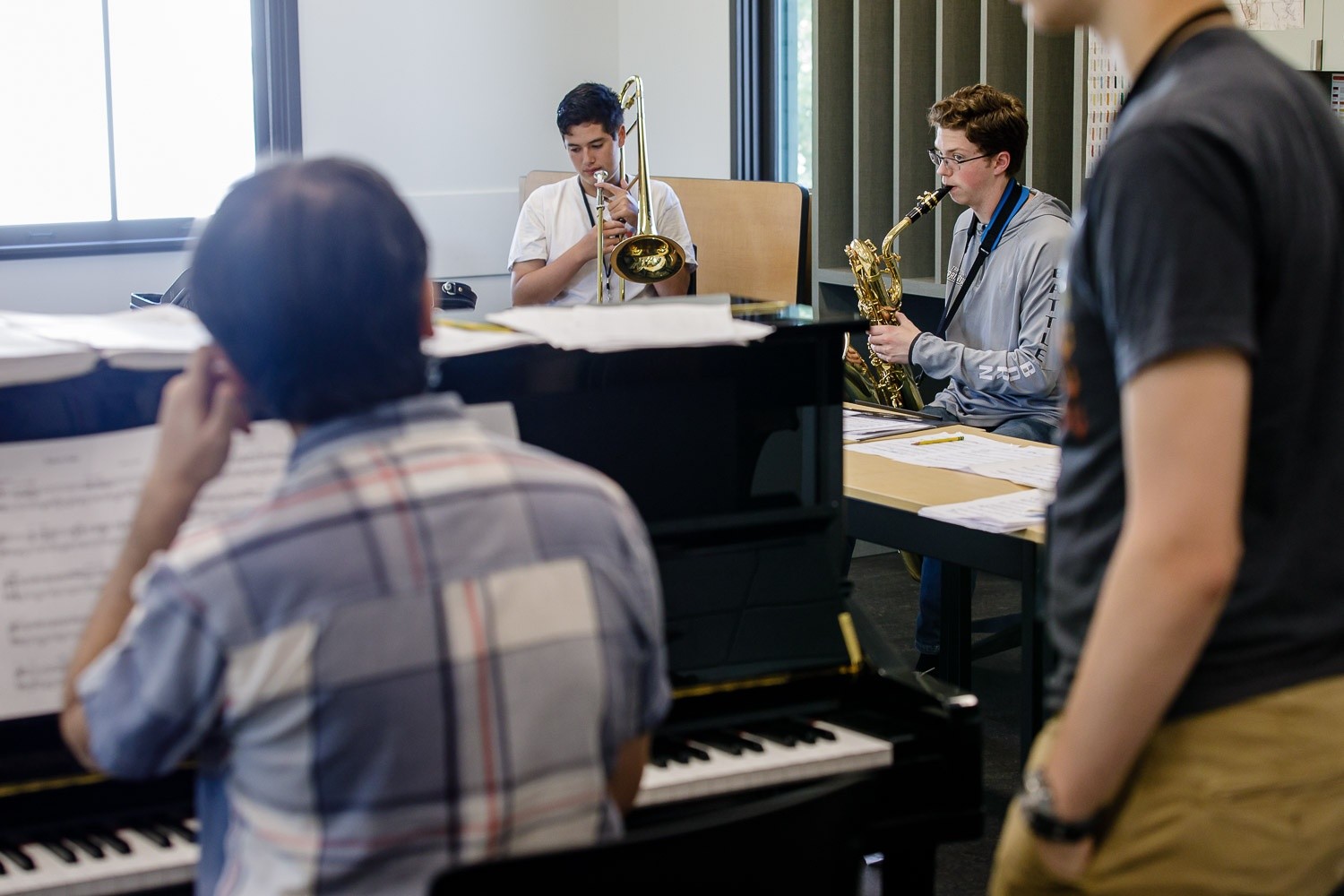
[424,769,942,895]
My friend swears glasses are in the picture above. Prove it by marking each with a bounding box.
[926,147,996,174]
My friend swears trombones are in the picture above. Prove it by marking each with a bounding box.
[592,75,685,301]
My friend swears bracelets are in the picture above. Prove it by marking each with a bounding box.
[1018,724,1113,842]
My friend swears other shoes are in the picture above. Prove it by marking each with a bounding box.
[896,546,922,583]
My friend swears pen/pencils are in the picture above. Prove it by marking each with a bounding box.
[910,436,964,445]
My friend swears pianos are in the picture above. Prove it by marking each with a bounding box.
[0,285,988,896]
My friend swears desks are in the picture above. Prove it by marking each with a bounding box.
[840,399,1064,776]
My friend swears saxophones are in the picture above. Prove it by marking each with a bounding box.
[839,184,954,412]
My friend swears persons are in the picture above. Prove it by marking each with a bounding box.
[868,82,1077,707]
[508,81,698,308]
[64,158,672,895]
[985,0,1344,896]
